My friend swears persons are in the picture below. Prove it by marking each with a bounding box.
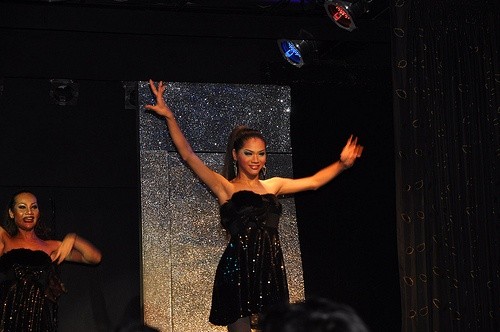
[0,192,102,332]
[261,300,369,332]
[145,79,363,332]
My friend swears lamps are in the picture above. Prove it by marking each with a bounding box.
[49,79,80,107]
[125,81,137,109]
[323,0,384,33]
[278,39,320,68]
